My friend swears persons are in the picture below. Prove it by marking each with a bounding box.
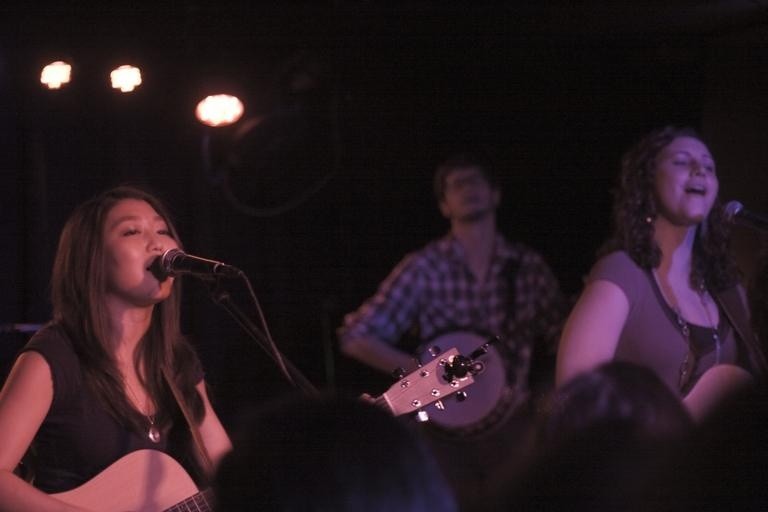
[335,155,574,471]
[557,125,764,426]
[0,186,235,512]
[460,358,716,512]
[210,399,460,512]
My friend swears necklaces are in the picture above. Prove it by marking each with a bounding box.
[125,378,160,444]
[656,270,721,371]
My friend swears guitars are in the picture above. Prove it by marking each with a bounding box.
[46,334,502,512]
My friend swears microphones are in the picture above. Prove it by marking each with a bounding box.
[719,199,768,234]
[158,245,241,279]
